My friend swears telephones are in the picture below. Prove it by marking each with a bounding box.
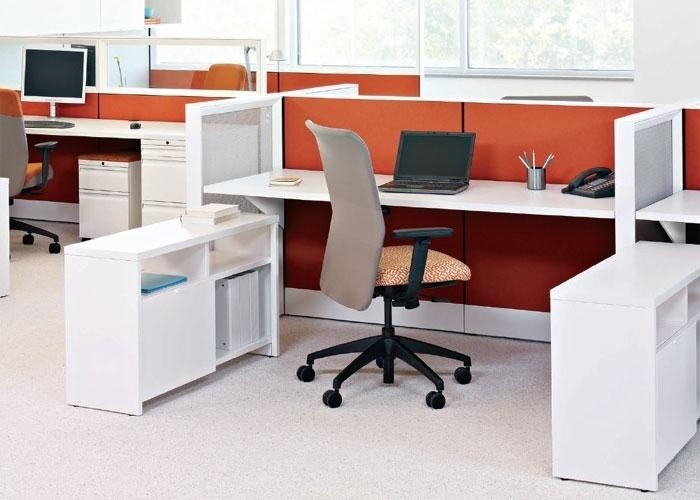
[562,167,615,198]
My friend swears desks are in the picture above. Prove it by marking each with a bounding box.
[203,166,614,229]
[636,190,700,243]
[22,115,261,242]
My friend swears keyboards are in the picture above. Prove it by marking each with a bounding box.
[23,120,75,129]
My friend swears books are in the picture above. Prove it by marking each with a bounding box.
[140,272,188,295]
[179,210,242,227]
[185,202,239,218]
[145,17,160,24]
[270,176,302,186]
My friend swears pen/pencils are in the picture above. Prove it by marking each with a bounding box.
[518,149,555,169]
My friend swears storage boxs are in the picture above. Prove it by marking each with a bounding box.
[215,271,259,351]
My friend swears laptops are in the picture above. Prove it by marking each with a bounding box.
[378,129,476,195]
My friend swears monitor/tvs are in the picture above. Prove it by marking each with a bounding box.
[71,43,96,86]
[19,46,86,104]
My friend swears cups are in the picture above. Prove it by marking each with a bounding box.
[527,166,546,191]
[144,7,153,18]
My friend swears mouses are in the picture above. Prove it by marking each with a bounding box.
[129,122,141,129]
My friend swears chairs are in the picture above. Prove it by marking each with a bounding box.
[0,89,61,254]
[204,64,246,91]
[299,120,471,413]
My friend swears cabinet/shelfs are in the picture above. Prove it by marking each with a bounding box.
[63,212,282,416]
[550,240,700,491]
